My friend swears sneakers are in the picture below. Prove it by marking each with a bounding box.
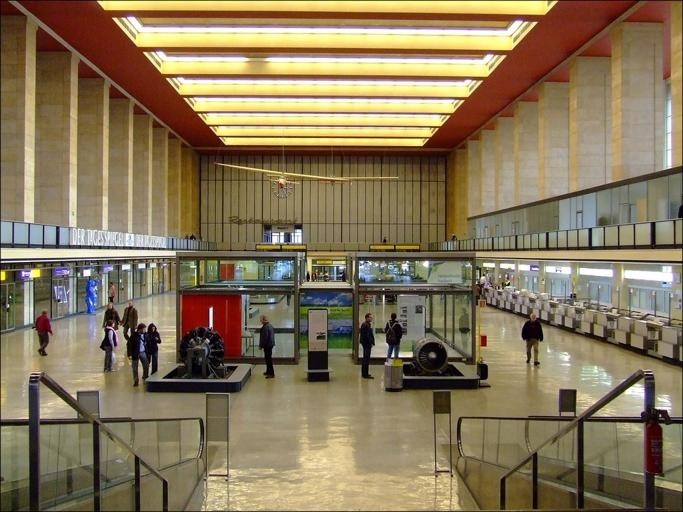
[39,349,48,355]
[363,375,373,379]
[527,360,540,366]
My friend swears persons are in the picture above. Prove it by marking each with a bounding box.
[451,233,457,241]
[306,270,346,282]
[36,311,53,356]
[190,233,196,240]
[385,313,402,358]
[258,316,275,378]
[476,274,486,305]
[459,308,469,350]
[360,314,375,378]
[100,282,161,386]
[383,237,387,243]
[522,314,543,365]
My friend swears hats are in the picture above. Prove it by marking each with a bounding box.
[261,316,268,322]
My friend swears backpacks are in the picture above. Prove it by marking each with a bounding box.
[386,321,399,344]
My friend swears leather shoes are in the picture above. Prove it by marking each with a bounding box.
[264,373,274,378]
